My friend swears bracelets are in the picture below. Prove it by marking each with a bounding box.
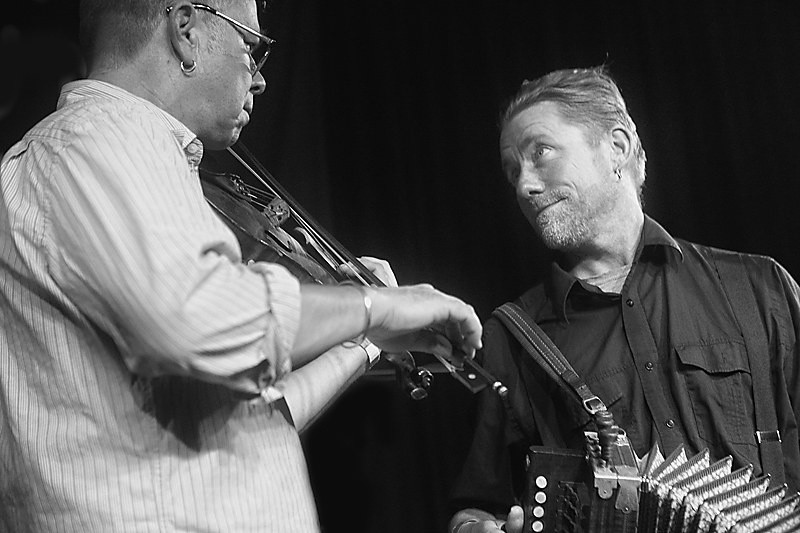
[336,280,372,350]
[347,335,381,372]
[453,518,478,533]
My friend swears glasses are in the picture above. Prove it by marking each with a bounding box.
[166,2,277,79]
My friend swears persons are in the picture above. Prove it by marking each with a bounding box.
[0,0,485,533]
[444,63,800,533]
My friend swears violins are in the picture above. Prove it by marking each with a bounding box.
[194,162,434,400]
